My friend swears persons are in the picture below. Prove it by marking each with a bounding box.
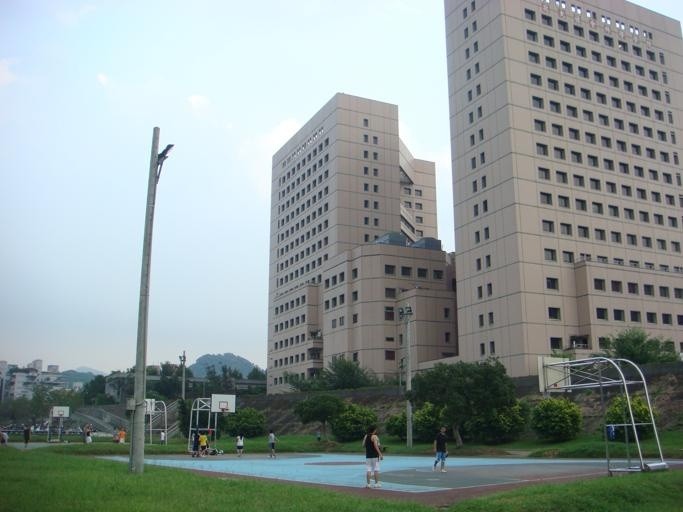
[85,427,91,444]
[113,425,126,444]
[433,427,448,473]
[269,430,275,457]
[198,432,209,455]
[317,429,320,441]
[236,434,244,456]
[362,425,383,488]
[24,425,30,448]
[191,432,199,457]
[161,430,165,445]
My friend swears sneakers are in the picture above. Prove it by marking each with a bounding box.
[440,469,447,472]
[432,467,436,471]
[374,484,381,487]
[365,484,370,488]
